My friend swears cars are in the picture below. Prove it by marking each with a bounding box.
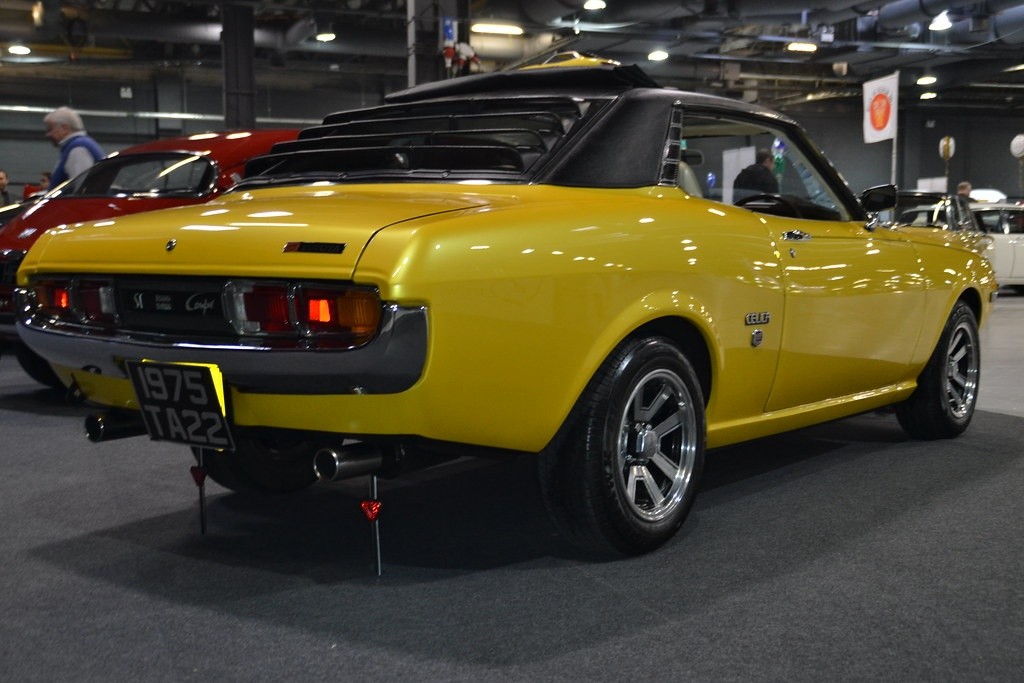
[893,188,986,232]
[0,128,303,388]
[12,57,999,562]
[0,188,48,227]
[895,202,1024,295]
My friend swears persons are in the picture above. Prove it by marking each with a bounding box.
[44,106,105,192]
[0,169,23,208]
[951,180,987,235]
[24,172,53,203]
[733,149,779,207]
